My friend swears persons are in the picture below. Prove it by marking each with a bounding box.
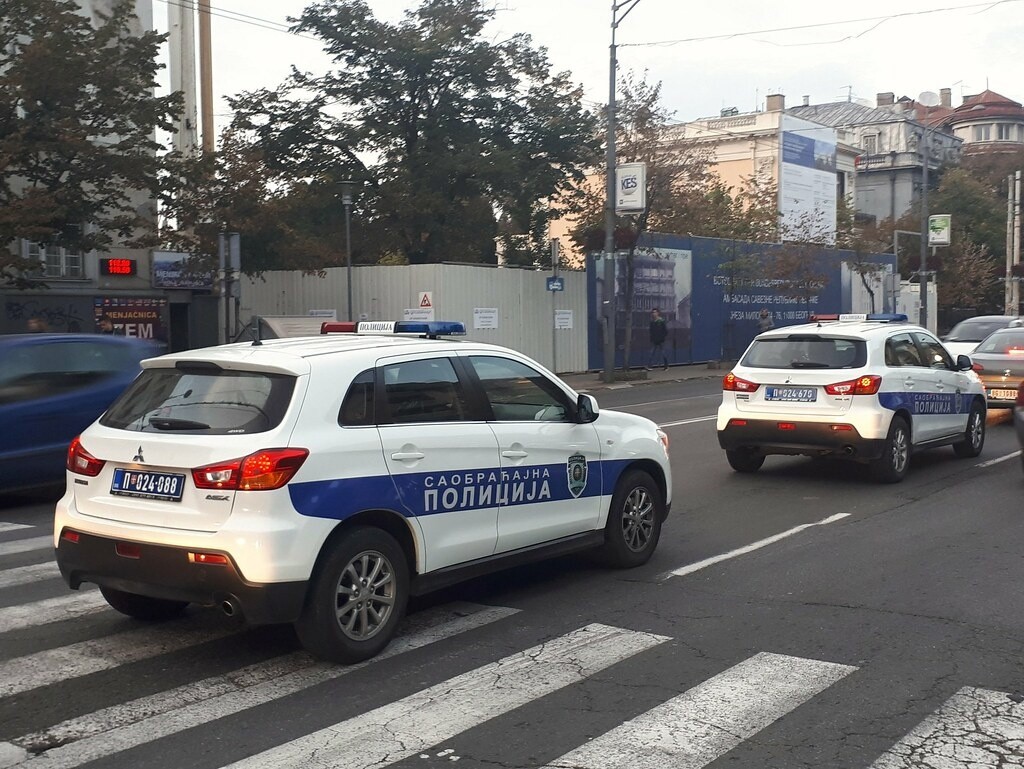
[26,316,53,333]
[644,308,670,372]
[98,314,121,337]
[755,312,775,333]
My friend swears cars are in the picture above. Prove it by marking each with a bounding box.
[934,314,1021,368]
[966,320,1023,412]
[1,335,167,508]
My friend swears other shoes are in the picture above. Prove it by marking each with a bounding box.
[648,368,653,371]
[663,367,669,371]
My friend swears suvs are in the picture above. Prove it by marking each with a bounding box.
[53,319,673,668]
[716,314,988,484]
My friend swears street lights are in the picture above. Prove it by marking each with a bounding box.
[336,179,360,324]
[919,103,986,329]
